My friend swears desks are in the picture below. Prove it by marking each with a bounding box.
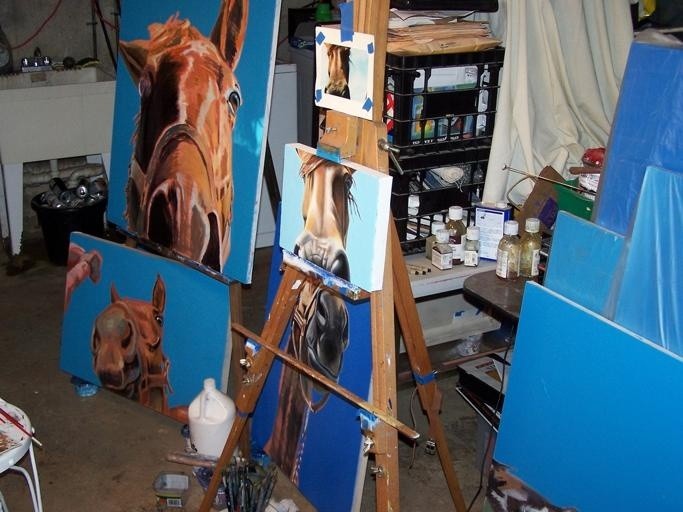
[0,68,116,272]
[390,245,543,385]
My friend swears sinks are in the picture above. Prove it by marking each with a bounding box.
[0,67,117,165]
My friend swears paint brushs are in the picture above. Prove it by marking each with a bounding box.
[405,264,431,276]
[222,456,279,512]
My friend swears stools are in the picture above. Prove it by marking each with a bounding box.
[1,397,47,512]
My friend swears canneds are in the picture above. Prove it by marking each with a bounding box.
[40,177,105,208]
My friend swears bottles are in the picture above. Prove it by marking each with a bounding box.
[425,205,481,271]
[521,218,541,276]
[495,219,520,281]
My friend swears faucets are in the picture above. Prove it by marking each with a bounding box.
[32,46,42,60]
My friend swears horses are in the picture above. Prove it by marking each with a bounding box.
[117,1,250,274]
[323,42,350,99]
[294,148,362,285]
[90,273,174,415]
[261,278,349,487]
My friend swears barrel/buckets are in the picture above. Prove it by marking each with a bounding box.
[187,379,239,466]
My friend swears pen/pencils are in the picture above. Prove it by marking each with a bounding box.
[0,408,43,447]
